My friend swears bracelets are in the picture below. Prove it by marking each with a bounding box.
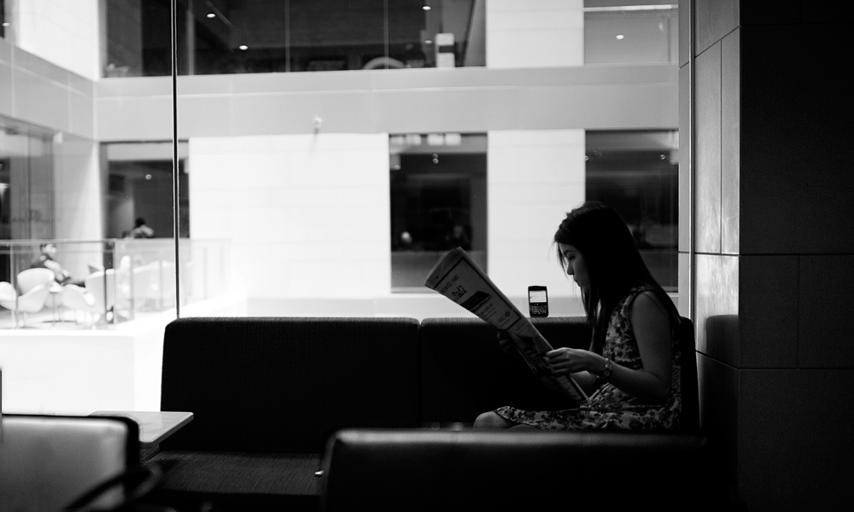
[599,356,612,383]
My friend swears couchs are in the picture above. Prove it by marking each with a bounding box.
[159,316,699,512]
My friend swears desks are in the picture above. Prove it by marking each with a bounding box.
[89,410,194,448]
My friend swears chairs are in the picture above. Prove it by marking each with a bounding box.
[0,256,167,326]
[0,413,152,512]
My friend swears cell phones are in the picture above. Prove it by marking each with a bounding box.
[528,286,548,317]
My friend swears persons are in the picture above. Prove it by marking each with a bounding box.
[31,244,116,325]
[161,197,189,239]
[468,198,687,435]
[123,216,155,239]
